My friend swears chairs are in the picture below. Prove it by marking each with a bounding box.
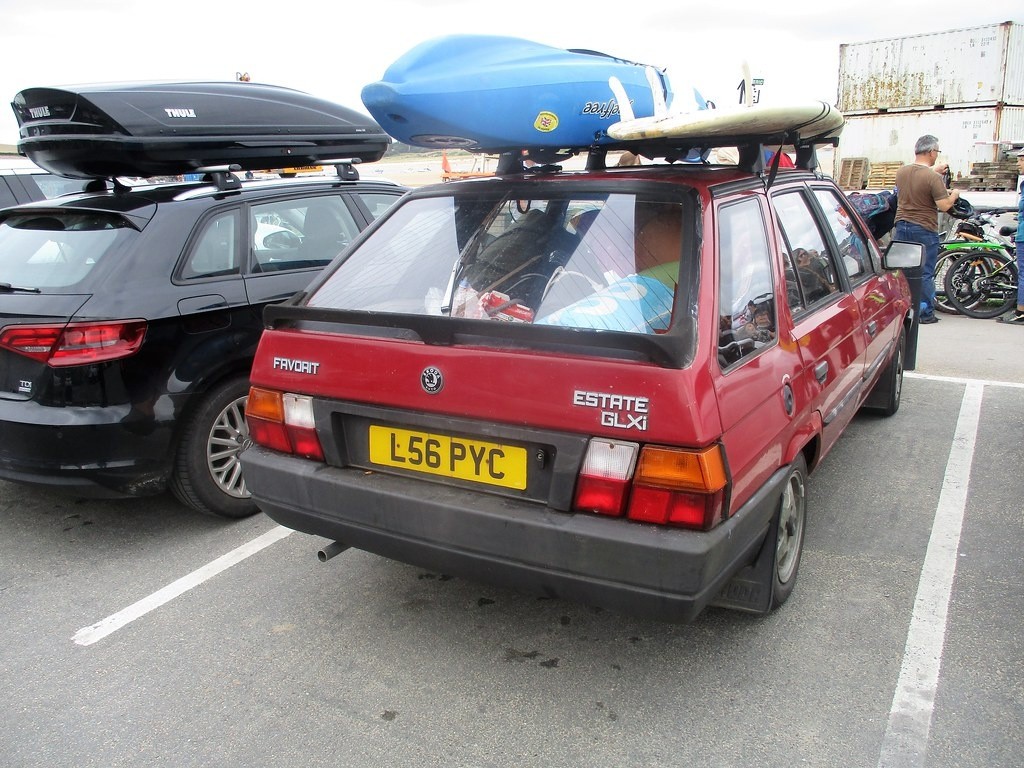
[211,211,258,270]
[296,206,350,260]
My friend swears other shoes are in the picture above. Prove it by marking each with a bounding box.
[918,314,938,324]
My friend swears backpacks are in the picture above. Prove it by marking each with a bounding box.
[458,209,674,334]
[846,188,898,241]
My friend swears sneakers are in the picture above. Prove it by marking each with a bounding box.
[996,309,1024,325]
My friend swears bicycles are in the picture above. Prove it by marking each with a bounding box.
[944,251,1019,319]
[933,209,1017,315]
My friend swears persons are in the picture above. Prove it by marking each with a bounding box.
[994,150,1024,326]
[891,135,961,324]
[717,218,870,364]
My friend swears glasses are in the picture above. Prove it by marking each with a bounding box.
[933,150,941,154]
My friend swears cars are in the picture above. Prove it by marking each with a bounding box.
[239,34,926,627]
[0,81,419,521]
[0,159,143,210]
